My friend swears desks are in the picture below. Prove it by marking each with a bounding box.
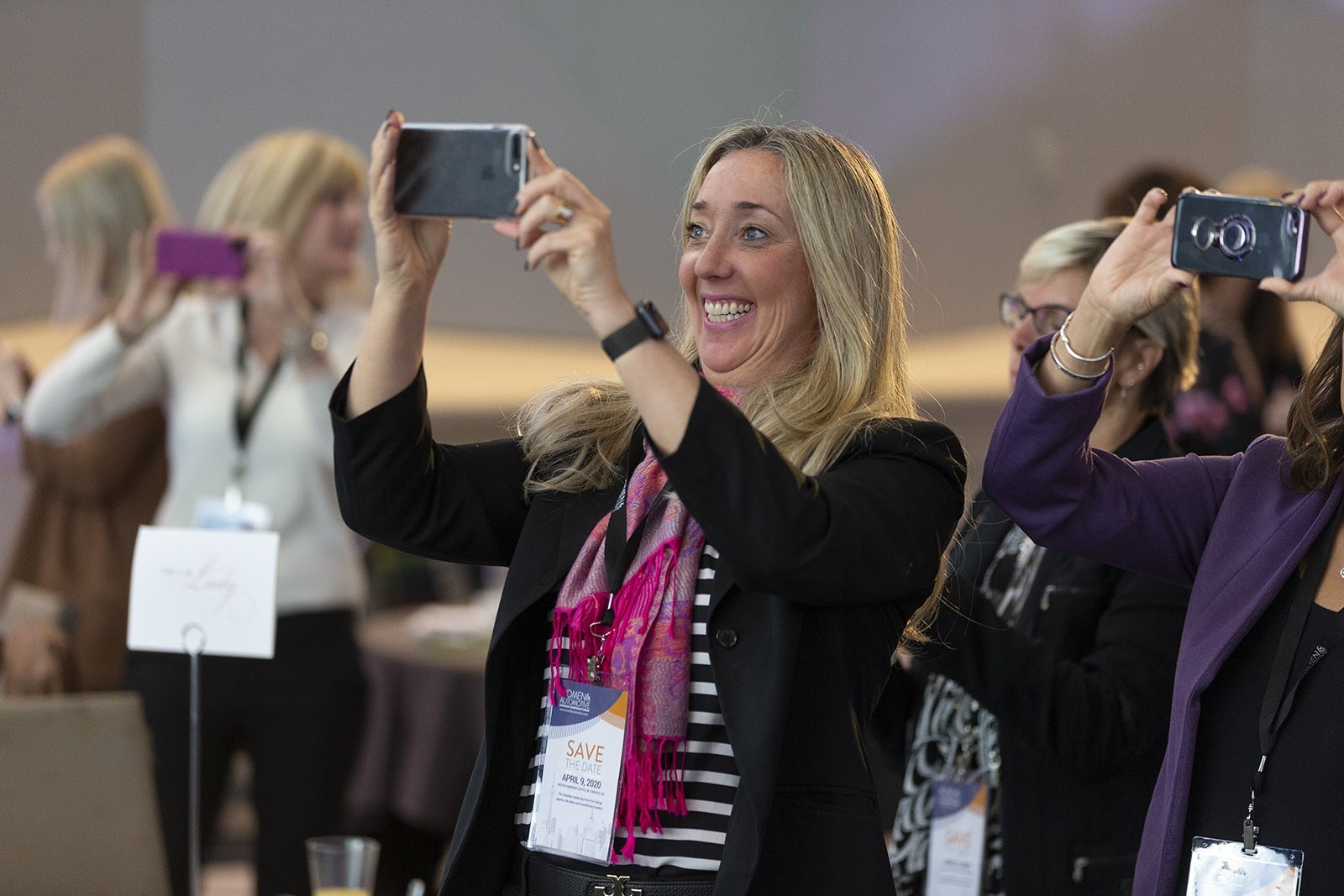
[342,564,510,896]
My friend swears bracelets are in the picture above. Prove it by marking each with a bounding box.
[1050,330,1110,379]
[1060,310,1114,362]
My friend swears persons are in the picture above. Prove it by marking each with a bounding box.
[0,118,1344,896]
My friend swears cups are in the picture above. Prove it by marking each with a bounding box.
[309,837,379,896]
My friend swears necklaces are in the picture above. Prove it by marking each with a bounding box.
[1340,567,1344,579]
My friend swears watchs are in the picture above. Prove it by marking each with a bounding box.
[601,300,668,361]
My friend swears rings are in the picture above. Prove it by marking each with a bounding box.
[553,200,575,224]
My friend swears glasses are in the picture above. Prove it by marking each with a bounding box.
[996,292,1073,338]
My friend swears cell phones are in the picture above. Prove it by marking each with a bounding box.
[392,124,534,220]
[156,230,250,280]
[1170,192,1310,283]
